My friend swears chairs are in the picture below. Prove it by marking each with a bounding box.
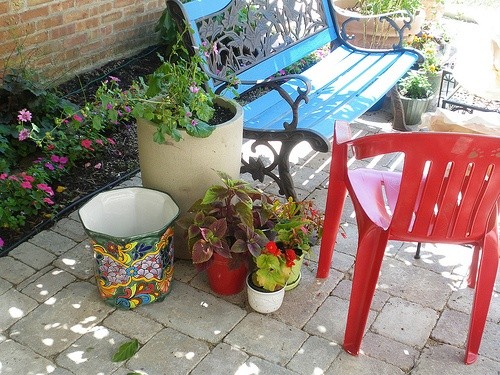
[318,120,500,366]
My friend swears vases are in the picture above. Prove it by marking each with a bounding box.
[77,186,181,313]
[426,74,443,86]
[137,99,244,260]
[244,272,286,315]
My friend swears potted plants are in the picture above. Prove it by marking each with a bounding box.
[331,0,425,49]
[396,77,432,125]
[272,201,327,289]
[181,176,261,294]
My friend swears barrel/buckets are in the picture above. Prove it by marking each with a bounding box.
[78,187,182,311]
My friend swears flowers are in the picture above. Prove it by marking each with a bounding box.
[17,25,245,153]
[422,50,442,73]
[259,242,299,288]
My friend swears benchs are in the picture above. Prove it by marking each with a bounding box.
[166,0,423,211]
